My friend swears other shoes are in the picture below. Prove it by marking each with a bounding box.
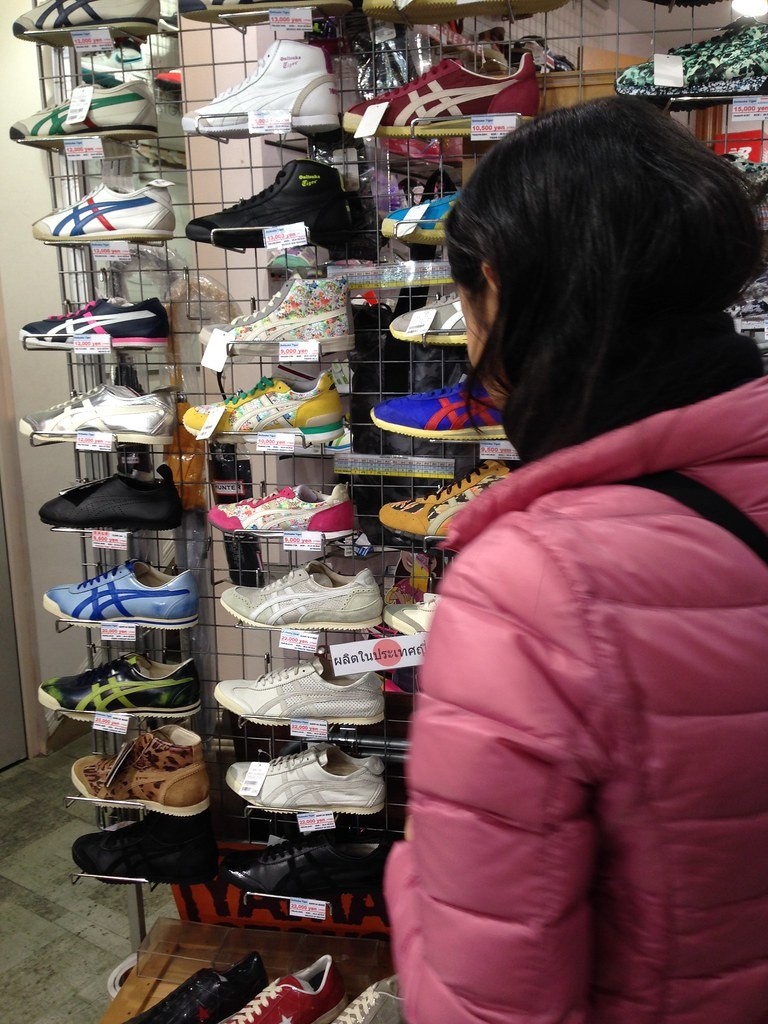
[220,830,393,902]
[71,811,221,886]
[362,1,569,25]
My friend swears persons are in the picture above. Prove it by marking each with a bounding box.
[382,95,768,1024]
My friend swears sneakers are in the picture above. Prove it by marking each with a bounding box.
[389,292,469,345]
[342,52,540,138]
[39,464,182,531]
[15,382,177,445]
[213,656,388,728]
[9,78,160,148]
[377,459,514,546]
[206,480,362,536]
[70,725,212,817]
[179,1,355,25]
[613,15,768,111]
[198,272,356,357]
[226,741,388,815]
[31,183,177,241]
[181,39,341,133]
[42,558,201,630]
[381,190,462,245]
[219,559,386,630]
[20,296,170,349]
[12,0,162,46]
[186,158,352,249]
[383,592,440,635]
[37,652,204,718]
[182,368,346,442]
[121,949,405,1024]
[369,371,507,439]
